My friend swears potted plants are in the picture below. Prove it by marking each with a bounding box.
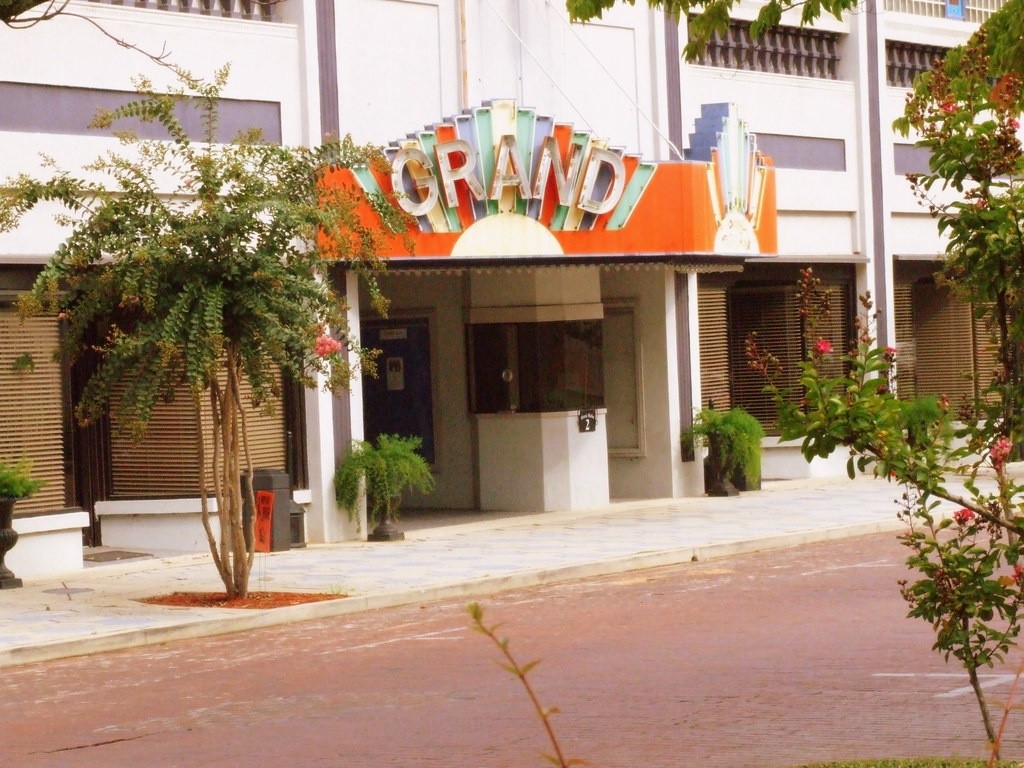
[333,432,438,542]
[681,402,768,496]
[0,445,55,589]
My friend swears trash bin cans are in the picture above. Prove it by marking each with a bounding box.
[240,466,293,555]
